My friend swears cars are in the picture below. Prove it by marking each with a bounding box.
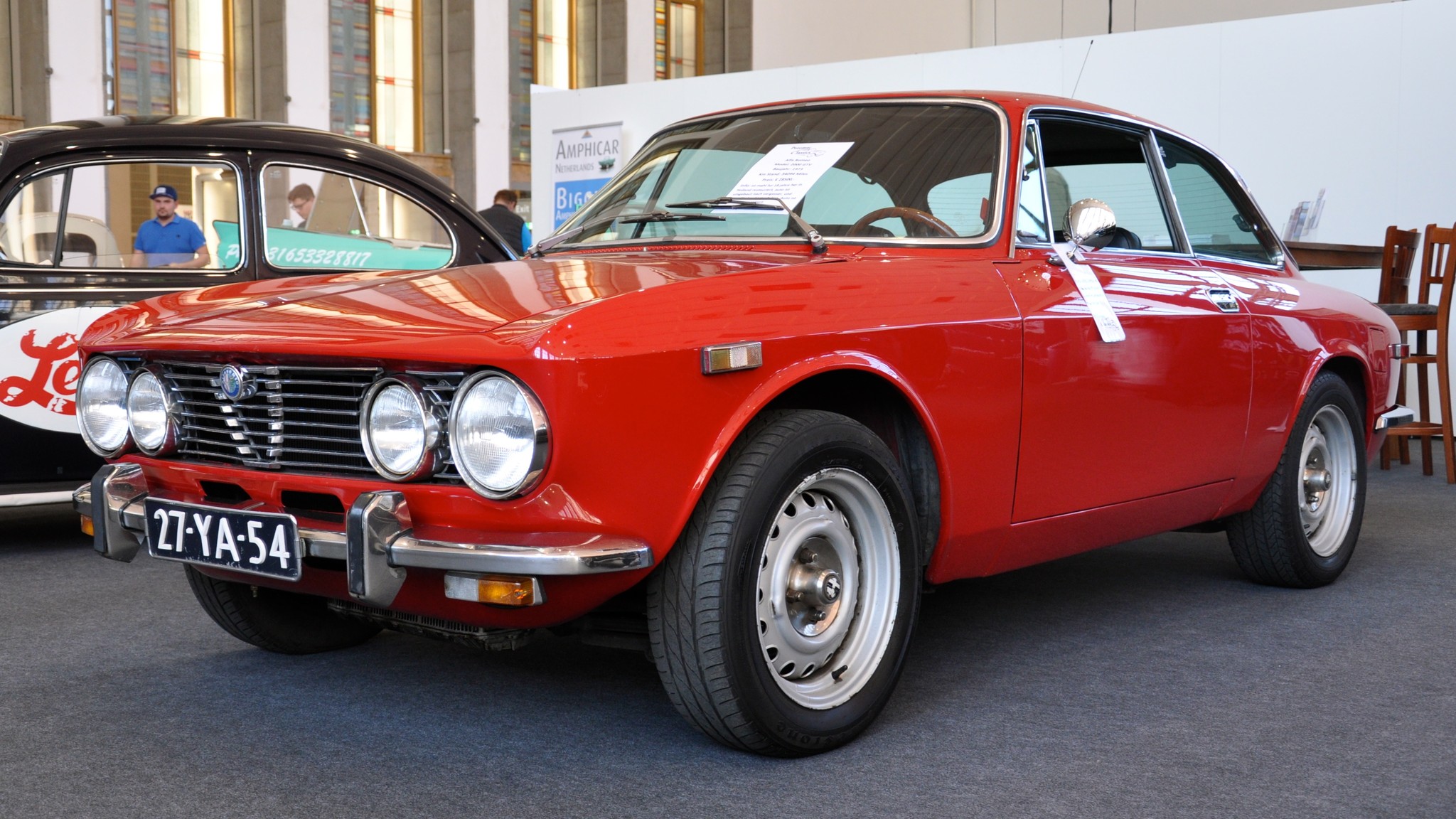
[73,91,1413,762]
[0,114,519,506]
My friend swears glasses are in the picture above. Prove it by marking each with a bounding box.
[291,200,310,210]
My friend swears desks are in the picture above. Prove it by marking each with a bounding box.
[1142,241,1399,271]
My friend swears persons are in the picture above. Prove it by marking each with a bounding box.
[287,184,315,228]
[478,190,532,257]
[131,184,211,268]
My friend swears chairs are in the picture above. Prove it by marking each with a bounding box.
[780,223,892,238]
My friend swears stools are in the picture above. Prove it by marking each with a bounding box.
[1378,226,1422,470]
[1374,223,1456,486]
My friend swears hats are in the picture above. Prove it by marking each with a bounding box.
[149,185,178,201]
